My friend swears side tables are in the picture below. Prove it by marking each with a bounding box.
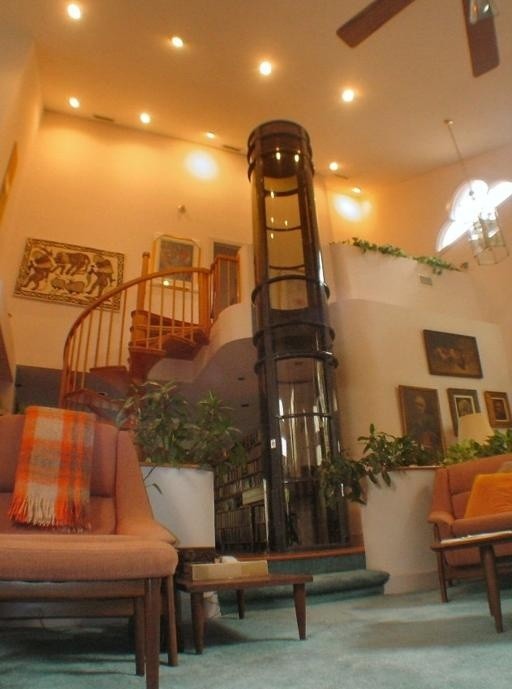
[174,575,313,654]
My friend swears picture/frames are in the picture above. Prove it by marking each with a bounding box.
[149,234,201,292]
[399,330,512,459]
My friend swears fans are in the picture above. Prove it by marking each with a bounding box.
[336,0,499,76]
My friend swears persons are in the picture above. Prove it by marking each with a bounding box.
[436,345,466,369]
[90,255,114,300]
[409,394,440,448]
[24,248,52,290]
[459,398,471,416]
[493,400,506,420]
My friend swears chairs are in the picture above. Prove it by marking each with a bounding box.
[0,414,178,689]
[427,453,512,603]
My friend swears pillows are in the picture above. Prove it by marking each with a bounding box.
[464,473,512,519]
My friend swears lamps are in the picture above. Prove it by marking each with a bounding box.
[444,120,510,266]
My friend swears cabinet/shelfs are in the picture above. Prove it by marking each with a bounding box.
[214,429,266,554]
